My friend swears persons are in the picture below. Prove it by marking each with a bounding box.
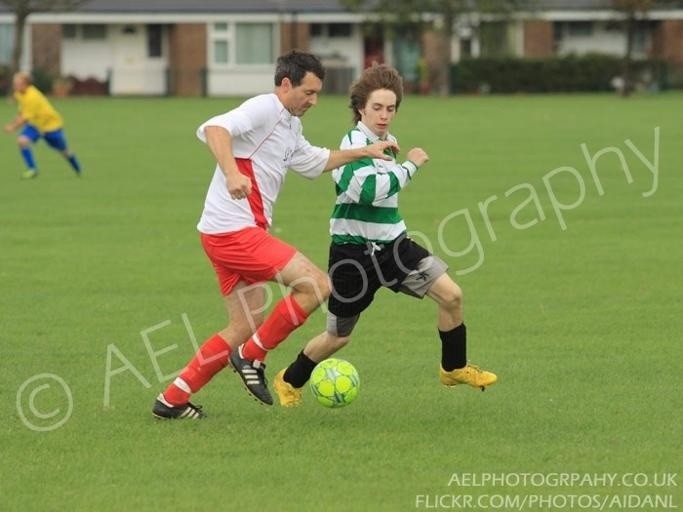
[2,70,83,180]
[150,47,403,424]
[270,59,499,409]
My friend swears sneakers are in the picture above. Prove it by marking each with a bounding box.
[271,368,302,406]
[439,362,498,388]
[153,393,208,420]
[228,344,274,407]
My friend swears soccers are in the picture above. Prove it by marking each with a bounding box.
[309,357,361,409]
